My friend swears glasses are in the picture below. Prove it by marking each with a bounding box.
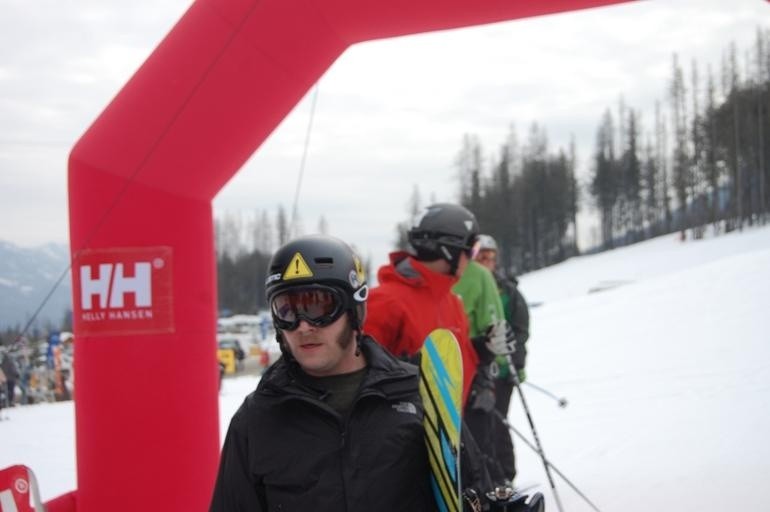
[269,283,352,331]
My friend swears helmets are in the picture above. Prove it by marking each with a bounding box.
[264,235,368,331]
[406,201,481,260]
[479,233,498,250]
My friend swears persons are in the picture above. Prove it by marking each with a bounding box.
[207,234,492,510]
[472,233,529,420]
[362,204,517,483]
[0,328,74,411]
[446,253,510,413]
[232,338,245,371]
